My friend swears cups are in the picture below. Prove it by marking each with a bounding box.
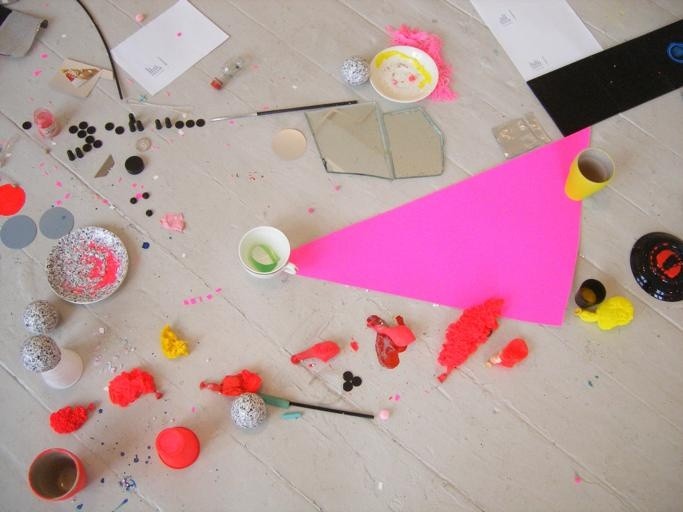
[155,427,201,471]
[564,147,618,203]
[26,447,90,504]
[238,223,301,282]
[573,279,606,312]
[40,349,84,390]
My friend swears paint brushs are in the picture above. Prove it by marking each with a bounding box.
[290,401,374,419]
[262,396,289,407]
[282,413,300,419]
[209,100,357,122]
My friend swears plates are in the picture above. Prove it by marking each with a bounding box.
[44,227,131,305]
[630,234,682,306]
[368,44,441,107]
